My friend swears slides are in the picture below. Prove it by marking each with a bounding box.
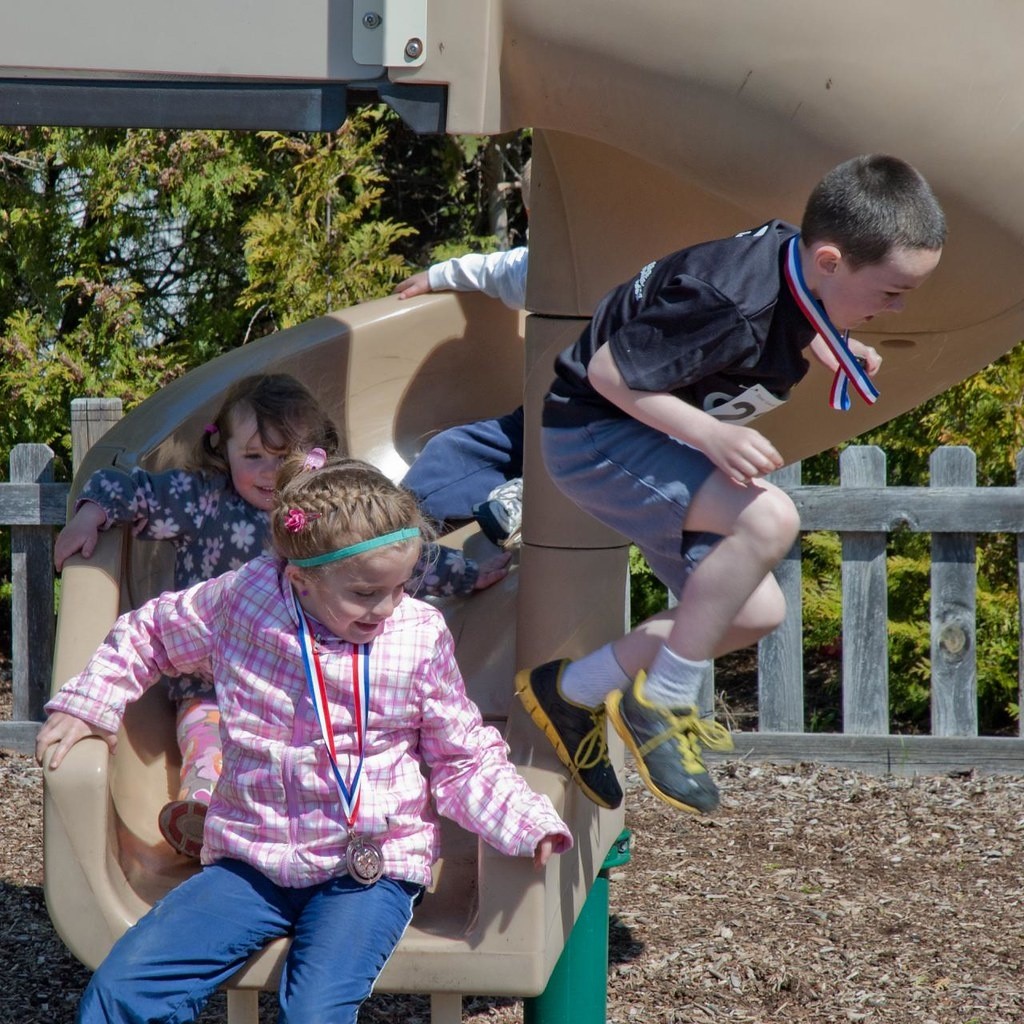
[40,1,1023,1024]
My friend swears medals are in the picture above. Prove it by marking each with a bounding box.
[349,841,385,884]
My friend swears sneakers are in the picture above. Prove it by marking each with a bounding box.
[471,473,527,550]
[158,797,209,861]
[514,656,627,810]
[605,667,736,817]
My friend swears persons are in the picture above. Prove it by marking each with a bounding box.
[394,159,530,552]
[51,378,511,859]
[513,153,947,819]
[34,442,576,1024]
